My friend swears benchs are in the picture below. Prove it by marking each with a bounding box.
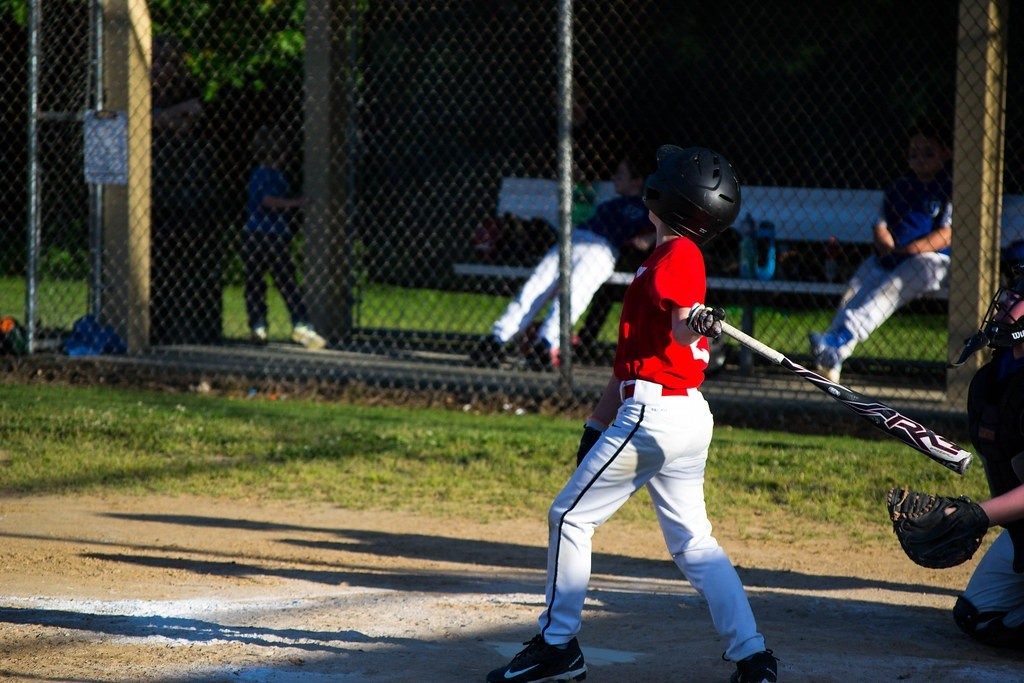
[453,177,1024,372]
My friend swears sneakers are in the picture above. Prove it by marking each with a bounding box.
[722,649,780,683]
[809,329,842,385]
[486,633,586,683]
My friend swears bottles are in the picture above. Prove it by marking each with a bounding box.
[291,324,326,350]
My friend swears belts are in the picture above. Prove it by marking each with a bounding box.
[624,383,688,399]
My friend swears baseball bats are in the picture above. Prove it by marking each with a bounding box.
[684,301,974,477]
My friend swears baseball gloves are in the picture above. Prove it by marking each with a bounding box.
[886,484,992,570]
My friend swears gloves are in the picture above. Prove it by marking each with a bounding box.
[576,423,601,468]
[686,302,725,345]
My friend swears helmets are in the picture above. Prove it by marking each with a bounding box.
[642,144,741,247]
[947,263,1024,369]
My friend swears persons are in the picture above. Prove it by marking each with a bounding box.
[470,152,658,374]
[809,123,955,383]
[887,257,1024,668]
[486,141,781,683]
[239,130,329,350]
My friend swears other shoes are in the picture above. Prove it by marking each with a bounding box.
[291,323,325,349]
[253,326,268,344]
[526,337,554,372]
[465,335,506,368]
